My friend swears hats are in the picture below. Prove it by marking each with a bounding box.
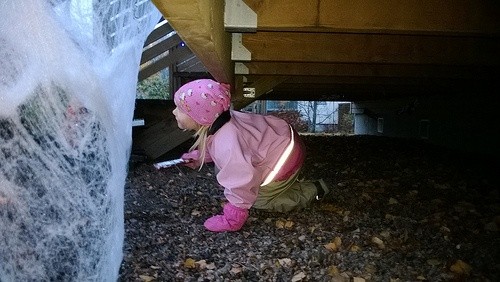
[173,79,234,125]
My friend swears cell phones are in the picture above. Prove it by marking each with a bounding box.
[153,158,192,170]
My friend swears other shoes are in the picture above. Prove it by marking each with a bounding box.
[315,179,329,203]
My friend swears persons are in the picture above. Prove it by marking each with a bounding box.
[172,79,331,233]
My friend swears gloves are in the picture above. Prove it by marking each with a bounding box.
[181,149,214,171]
[204,202,249,232]
